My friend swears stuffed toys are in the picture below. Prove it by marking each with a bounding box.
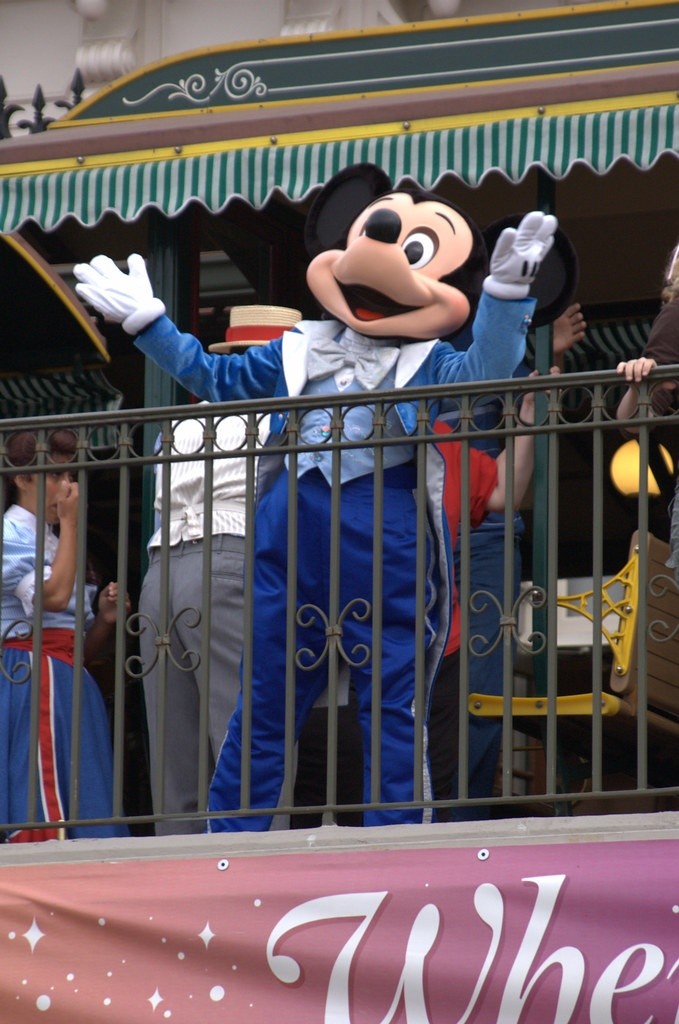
[73,161,561,840]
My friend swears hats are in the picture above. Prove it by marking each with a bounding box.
[209,305,302,354]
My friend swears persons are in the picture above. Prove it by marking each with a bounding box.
[617,241,679,579]
[138,302,587,837]
[1,428,130,843]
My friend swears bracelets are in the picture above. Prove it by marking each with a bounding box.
[518,417,533,427]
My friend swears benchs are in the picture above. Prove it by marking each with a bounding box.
[467,530,679,816]
